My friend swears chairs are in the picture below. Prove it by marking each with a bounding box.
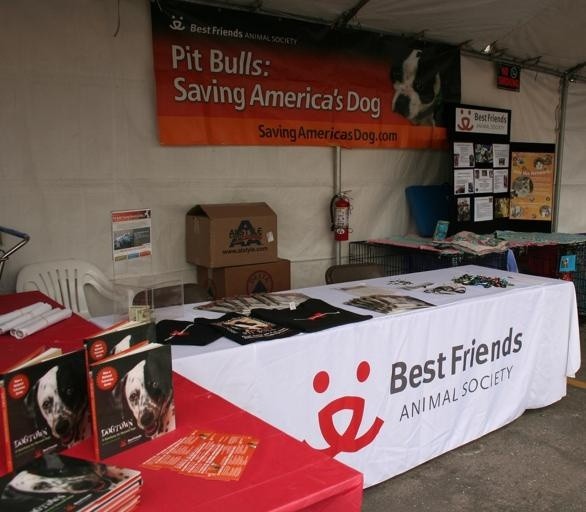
[16,259,132,330]
[133,280,212,309]
[326,264,385,284]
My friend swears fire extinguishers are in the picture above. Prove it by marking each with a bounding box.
[331,190,354,241]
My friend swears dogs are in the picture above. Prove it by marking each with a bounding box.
[389,41,461,128]
[108,354,172,439]
[4,453,112,493]
[23,360,88,448]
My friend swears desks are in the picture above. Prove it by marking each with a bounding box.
[0,291,363,512]
[349,241,586,326]
[87,264,581,489]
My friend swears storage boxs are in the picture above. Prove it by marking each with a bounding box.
[197,258,290,297]
[186,202,277,269]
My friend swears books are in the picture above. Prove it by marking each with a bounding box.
[0,318,178,512]
